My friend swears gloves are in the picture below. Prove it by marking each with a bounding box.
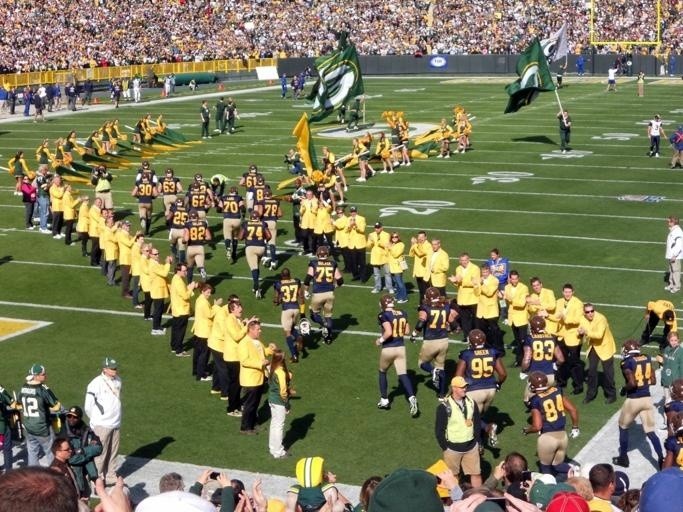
[410,331,418,344]
[304,291,311,300]
[336,277,344,287]
[570,427,580,439]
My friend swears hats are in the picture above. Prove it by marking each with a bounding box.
[368,468,444,512]
[101,357,118,370]
[529,477,591,512]
[66,406,84,419]
[374,222,382,227]
[451,376,469,389]
[350,206,357,212]
[299,486,324,510]
[613,467,683,512]
[664,310,675,327]
[28,364,45,376]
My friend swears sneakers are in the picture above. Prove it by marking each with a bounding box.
[557,383,617,404]
[665,285,681,294]
[13,190,167,336]
[408,396,418,415]
[612,457,630,468]
[251,287,262,299]
[326,185,348,216]
[432,368,440,388]
[354,138,470,182]
[170,349,295,459]
[290,336,303,363]
[200,269,207,283]
[321,327,332,345]
[202,127,235,138]
[486,423,498,447]
[226,248,231,260]
[261,256,278,271]
[371,287,409,303]
[376,401,390,410]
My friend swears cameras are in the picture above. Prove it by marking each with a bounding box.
[210,472,220,479]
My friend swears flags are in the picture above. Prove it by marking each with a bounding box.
[503,38,555,115]
[299,44,365,123]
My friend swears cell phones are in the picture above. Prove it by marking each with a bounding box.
[523,471,531,487]
[472,497,509,512]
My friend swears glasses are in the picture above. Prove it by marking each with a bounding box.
[585,309,594,314]
[392,236,399,239]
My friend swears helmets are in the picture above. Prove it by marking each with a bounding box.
[299,318,311,337]
[425,287,441,304]
[467,329,487,350]
[530,315,546,333]
[622,340,642,355]
[529,370,547,391]
[316,245,329,259]
[141,161,272,222]
[670,379,683,434]
[379,295,395,308]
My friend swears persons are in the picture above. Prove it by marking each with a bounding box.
[291,72,305,99]
[346,99,361,132]
[219,102,235,133]
[557,63,566,86]
[636,71,645,97]
[0,72,200,122]
[606,66,621,93]
[213,96,227,132]
[355,94,364,113]
[200,99,214,139]
[224,96,240,120]
[280,72,287,100]
